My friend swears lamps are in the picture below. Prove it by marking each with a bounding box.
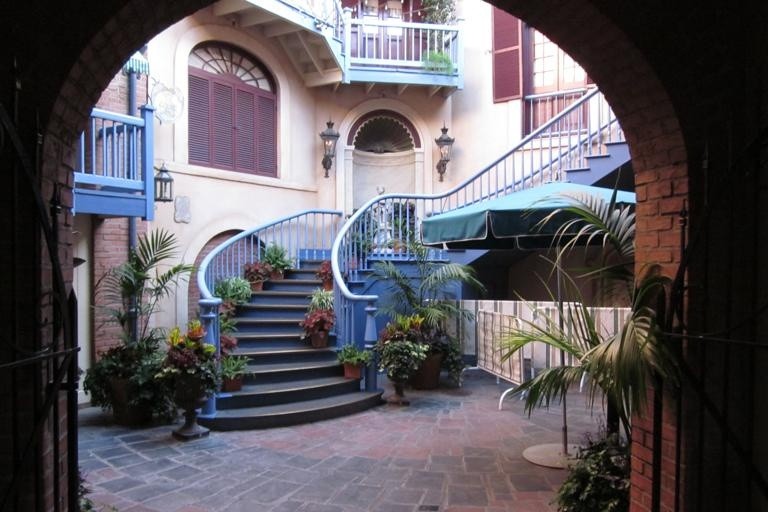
[155,163,175,203]
[435,120,455,182]
[319,114,340,178]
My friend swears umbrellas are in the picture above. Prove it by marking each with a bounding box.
[420,181,636,453]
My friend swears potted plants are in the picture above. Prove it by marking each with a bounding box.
[300,202,415,348]
[366,230,488,390]
[218,242,294,392]
[83,227,202,427]
[373,313,431,405]
[338,344,373,379]
[152,320,220,441]
